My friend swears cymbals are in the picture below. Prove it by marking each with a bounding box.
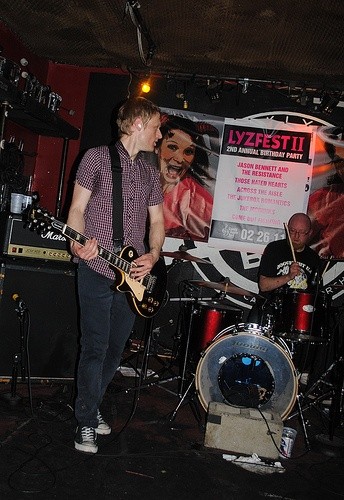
[187,279,264,299]
[158,249,213,265]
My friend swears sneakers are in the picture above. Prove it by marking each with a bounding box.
[94,411,111,435]
[73,423,98,454]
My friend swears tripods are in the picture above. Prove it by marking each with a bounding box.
[116,259,230,426]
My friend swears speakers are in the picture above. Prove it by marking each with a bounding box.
[0,263,84,384]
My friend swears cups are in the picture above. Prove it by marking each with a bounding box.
[11,193,24,215]
[279,427,296,460]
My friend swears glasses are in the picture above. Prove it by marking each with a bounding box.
[288,228,310,237]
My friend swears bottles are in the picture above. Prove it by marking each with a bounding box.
[22,176,33,211]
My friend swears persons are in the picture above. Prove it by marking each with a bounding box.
[64,97,166,453]
[258,212,324,300]
[151,116,212,240]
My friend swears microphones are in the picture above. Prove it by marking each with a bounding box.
[12,292,26,306]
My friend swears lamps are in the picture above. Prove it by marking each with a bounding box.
[207,82,225,106]
[318,93,340,117]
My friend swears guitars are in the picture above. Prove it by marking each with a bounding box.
[21,203,169,320]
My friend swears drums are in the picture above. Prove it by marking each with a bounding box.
[192,322,299,432]
[269,288,318,340]
[194,300,243,358]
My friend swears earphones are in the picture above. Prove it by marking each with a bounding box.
[137,124,142,130]
